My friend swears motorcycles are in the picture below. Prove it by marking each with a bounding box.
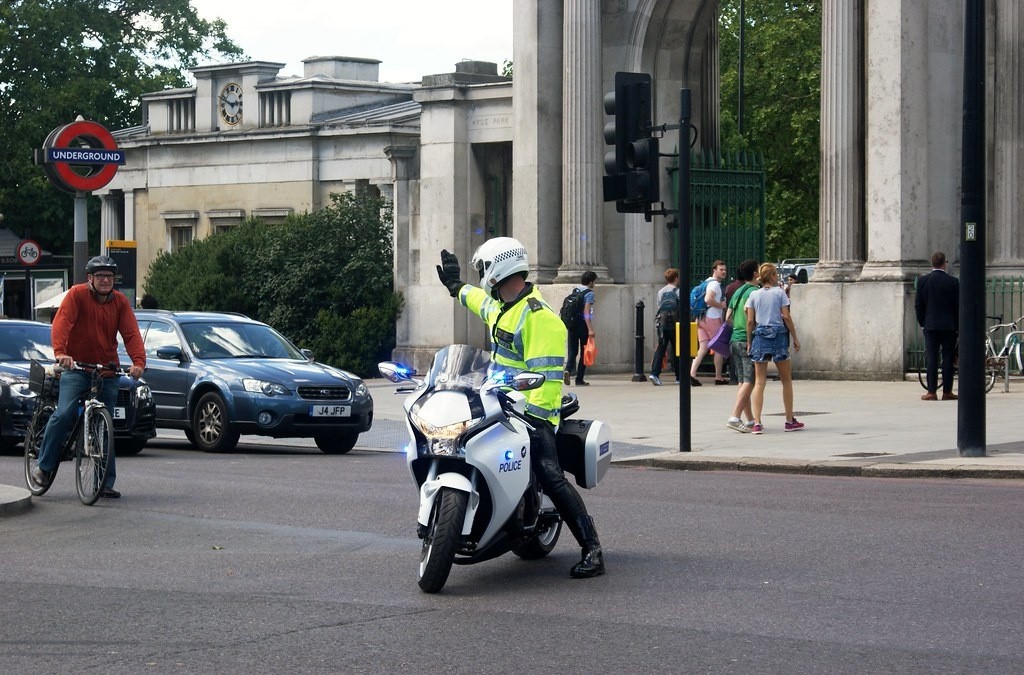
[373,344,618,587]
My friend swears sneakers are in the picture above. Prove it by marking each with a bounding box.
[753,423,765,434]
[727,417,752,433]
[649,374,662,386]
[744,420,754,430]
[784,417,805,432]
[675,380,680,385]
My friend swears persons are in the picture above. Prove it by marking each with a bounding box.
[914,252,959,400]
[691,261,745,386]
[649,269,680,385]
[785,274,798,298]
[745,263,804,434]
[435,237,605,577]
[726,261,763,433]
[564,271,598,385]
[140,295,158,309]
[32,256,146,498]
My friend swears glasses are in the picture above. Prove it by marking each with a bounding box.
[92,273,116,280]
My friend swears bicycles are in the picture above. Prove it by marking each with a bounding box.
[913,314,1024,397]
[24,361,132,506]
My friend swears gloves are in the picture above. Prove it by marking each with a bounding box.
[524,412,544,453]
[436,248,467,297]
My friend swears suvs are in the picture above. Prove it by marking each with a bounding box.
[118,310,374,453]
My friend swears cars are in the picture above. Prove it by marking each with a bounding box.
[0,319,159,455]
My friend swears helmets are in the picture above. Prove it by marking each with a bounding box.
[471,236,530,304]
[85,255,119,275]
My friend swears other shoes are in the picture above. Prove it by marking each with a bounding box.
[715,379,730,385]
[563,370,571,386]
[574,382,590,386]
[690,376,702,387]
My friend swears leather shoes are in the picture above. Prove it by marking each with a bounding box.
[942,391,959,400]
[32,461,50,488]
[920,392,937,400]
[92,486,121,498]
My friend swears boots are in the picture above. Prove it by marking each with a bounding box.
[568,515,605,579]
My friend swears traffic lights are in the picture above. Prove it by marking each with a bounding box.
[600,70,653,208]
[627,136,666,213]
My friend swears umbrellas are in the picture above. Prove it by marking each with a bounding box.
[33,290,69,309]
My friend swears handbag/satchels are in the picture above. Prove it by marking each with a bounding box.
[582,335,597,367]
[706,320,734,358]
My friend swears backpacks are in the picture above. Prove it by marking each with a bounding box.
[689,278,720,322]
[655,287,680,341]
[559,287,594,333]
